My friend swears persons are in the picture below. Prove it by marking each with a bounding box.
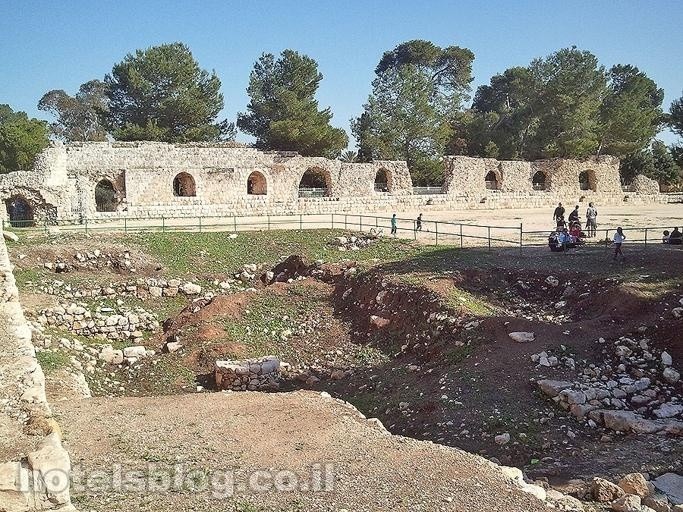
[391,214,396,234]
[663,230,670,243]
[670,227,682,244]
[612,227,626,258]
[416,213,423,231]
[548,201,597,252]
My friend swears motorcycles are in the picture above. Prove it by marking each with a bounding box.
[549,215,584,252]
